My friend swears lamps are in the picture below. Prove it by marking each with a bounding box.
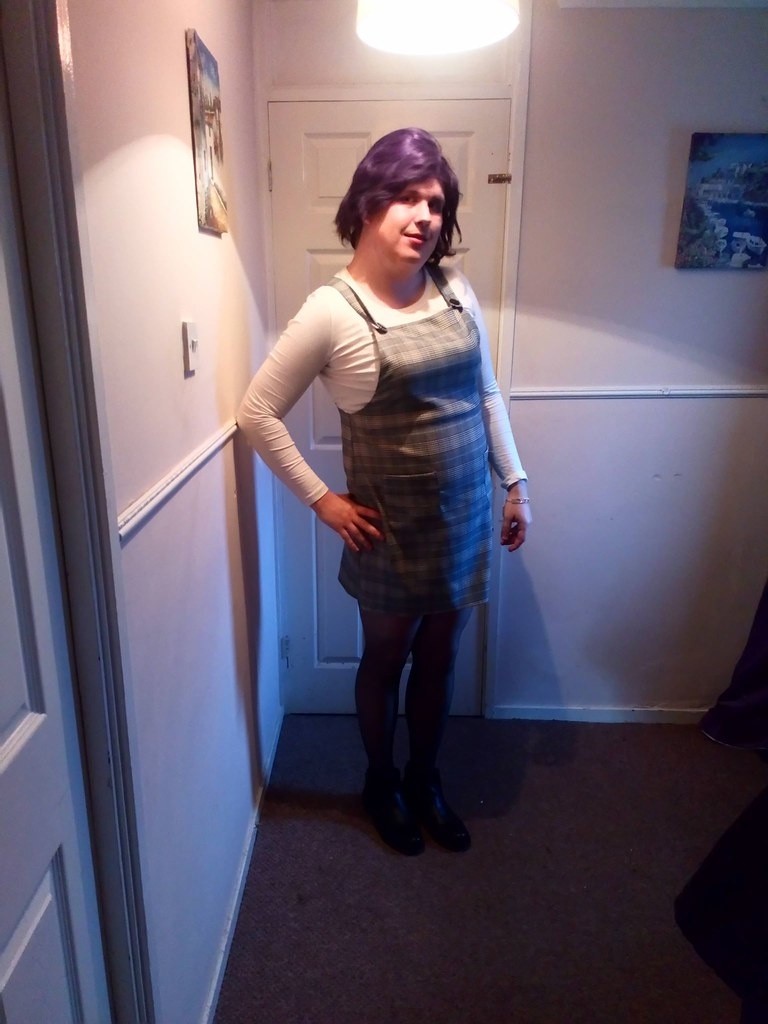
[355,0,524,53]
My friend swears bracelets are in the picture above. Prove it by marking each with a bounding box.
[504,496,531,504]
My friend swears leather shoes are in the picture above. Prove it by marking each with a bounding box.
[403,763,471,852]
[361,770,423,856]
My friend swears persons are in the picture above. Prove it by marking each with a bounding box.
[237,129,534,854]
